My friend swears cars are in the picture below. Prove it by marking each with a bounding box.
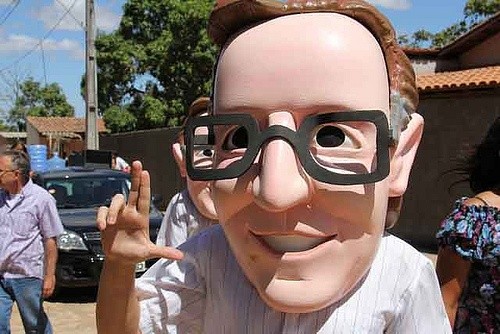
[32,169,165,302]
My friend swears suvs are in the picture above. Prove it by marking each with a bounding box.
[66,149,129,169]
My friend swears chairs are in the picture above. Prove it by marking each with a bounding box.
[72,182,91,195]
[48,185,67,200]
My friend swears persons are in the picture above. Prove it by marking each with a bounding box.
[0,150,65,334]
[154,92,221,255]
[90,0,453,333]
[433,117,500,334]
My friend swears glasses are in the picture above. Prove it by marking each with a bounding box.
[0,169,14,176]
